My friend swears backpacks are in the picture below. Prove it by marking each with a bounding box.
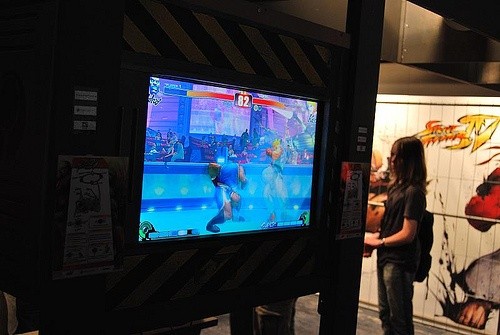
[413,210,434,282]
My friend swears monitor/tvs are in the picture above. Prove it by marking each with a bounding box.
[133,71,322,249]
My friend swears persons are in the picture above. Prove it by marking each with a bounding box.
[364,136,427,335]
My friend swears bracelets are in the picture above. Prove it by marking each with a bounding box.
[382,237,385,246]
